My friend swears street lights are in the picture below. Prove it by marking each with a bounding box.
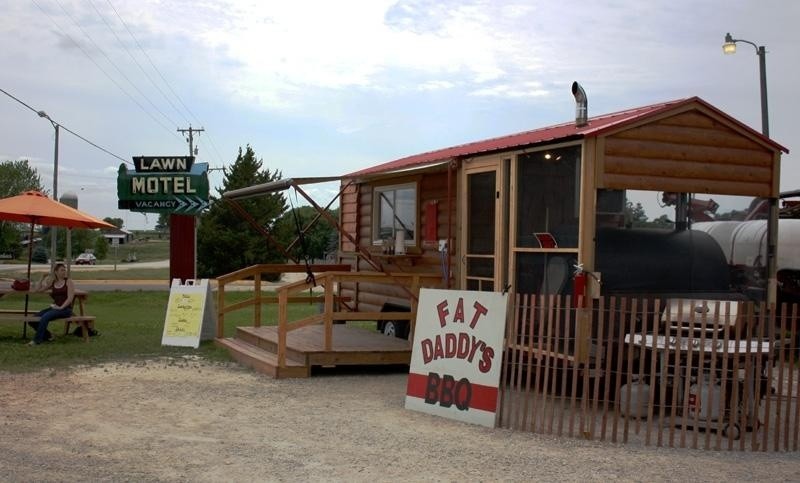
[723,32,771,139]
[37,109,59,276]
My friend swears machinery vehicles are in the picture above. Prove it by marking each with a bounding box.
[656,188,800,302]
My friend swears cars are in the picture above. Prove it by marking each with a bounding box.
[75,252,97,265]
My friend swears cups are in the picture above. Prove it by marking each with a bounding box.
[395,230,407,255]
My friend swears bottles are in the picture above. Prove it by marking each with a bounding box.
[382,244,394,255]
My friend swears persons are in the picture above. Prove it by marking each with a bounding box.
[27,262,76,346]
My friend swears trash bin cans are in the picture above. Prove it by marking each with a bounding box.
[319,295,347,325]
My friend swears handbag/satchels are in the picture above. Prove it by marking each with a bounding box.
[74,326,98,337]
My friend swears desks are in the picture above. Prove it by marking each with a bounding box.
[1,285,89,342]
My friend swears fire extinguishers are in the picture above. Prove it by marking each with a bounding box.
[568,263,603,313]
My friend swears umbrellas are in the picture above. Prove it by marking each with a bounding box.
[1,185,118,342]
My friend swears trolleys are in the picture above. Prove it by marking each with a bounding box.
[620,331,793,440]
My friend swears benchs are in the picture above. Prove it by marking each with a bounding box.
[0,314,99,342]
[1,307,40,315]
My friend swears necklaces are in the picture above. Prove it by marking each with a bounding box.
[53,280,64,287]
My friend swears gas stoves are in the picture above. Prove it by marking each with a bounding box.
[623,326,791,354]
